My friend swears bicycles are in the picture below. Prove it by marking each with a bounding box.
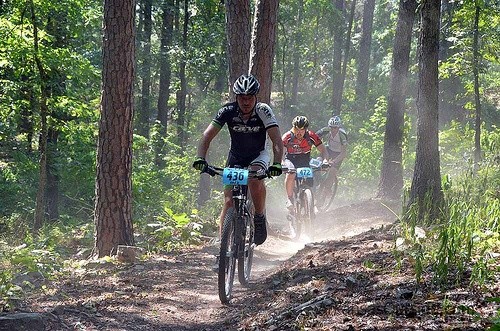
[197,163,291,303]
[310,165,341,211]
[275,164,328,242]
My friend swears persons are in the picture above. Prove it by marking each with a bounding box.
[281,116,329,216]
[315,116,348,207]
[193,74,283,272]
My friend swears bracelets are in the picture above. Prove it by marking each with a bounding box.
[331,160,337,165]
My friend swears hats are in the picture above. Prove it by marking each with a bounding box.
[232,74,260,95]
[328,116,341,127]
[291,116,309,129]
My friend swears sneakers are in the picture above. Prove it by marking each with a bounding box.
[253,215,267,244]
[286,197,294,208]
[212,251,229,268]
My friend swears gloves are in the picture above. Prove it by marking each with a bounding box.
[282,164,288,173]
[267,163,282,178]
[193,156,208,170]
[322,159,329,168]
